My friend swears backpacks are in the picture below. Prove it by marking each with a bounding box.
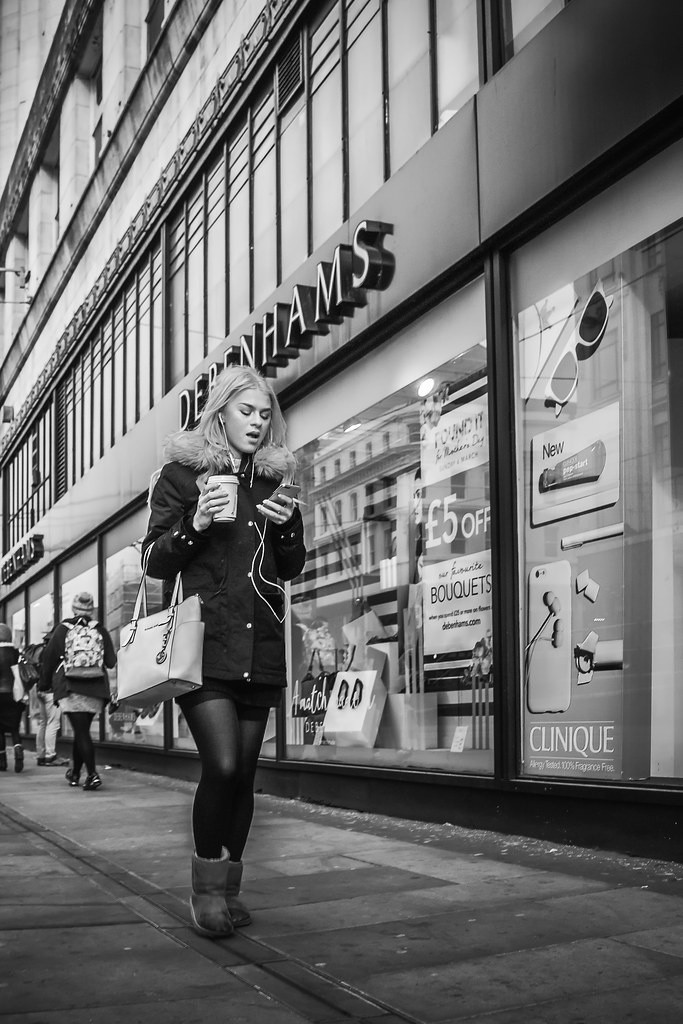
[60,620,105,677]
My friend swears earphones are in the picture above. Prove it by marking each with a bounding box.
[218,411,232,427]
[525,589,565,653]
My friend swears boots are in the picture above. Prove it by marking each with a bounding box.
[0,751,7,770]
[225,858,252,928]
[14,745,24,772]
[189,844,235,938]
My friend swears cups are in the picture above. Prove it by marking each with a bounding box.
[207,475,240,522]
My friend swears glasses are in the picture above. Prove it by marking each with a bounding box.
[545,278,614,417]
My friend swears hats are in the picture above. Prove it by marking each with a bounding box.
[72,592,94,616]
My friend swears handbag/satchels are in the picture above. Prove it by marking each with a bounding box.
[293,648,330,717]
[289,600,392,749]
[11,650,39,706]
[115,541,205,709]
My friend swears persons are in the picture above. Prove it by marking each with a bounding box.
[38,591,117,790]
[0,623,71,773]
[139,364,305,937]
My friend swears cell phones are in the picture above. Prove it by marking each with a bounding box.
[258,484,301,513]
[528,561,572,713]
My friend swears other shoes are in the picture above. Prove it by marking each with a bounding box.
[65,769,80,786]
[84,773,102,790]
[45,756,70,766]
[37,760,45,765]
